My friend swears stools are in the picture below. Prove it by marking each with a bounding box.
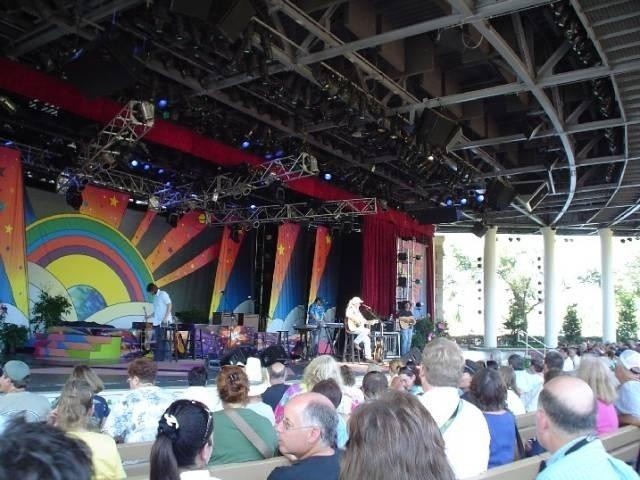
[160,327,175,356]
[183,329,203,360]
[277,330,290,362]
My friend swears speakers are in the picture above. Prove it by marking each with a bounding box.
[167,0,211,22]
[420,107,457,148]
[488,178,516,209]
[417,207,458,225]
[212,0,256,44]
[471,222,488,238]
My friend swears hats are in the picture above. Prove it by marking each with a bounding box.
[243,358,270,397]
[352,297,364,305]
[620,349,640,374]
[4,360,31,382]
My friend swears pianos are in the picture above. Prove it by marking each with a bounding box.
[293,323,344,329]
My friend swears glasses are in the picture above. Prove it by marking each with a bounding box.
[279,417,318,430]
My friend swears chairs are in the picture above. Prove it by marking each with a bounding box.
[343,317,364,364]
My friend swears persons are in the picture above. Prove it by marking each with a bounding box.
[1,337,639,478]
[344,296,375,363]
[397,299,419,357]
[147,282,173,362]
[308,296,324,358]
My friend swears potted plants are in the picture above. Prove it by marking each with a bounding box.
[438,319,448,329]
[558,304,583,358]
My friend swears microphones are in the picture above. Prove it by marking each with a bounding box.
[142,306,147,316]
[361,304,371,310]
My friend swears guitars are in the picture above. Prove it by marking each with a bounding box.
[172,316,187,354]
[375,323,388,360]
[345,317,380,333]
[140,308,154,351]
[399,316,421,328]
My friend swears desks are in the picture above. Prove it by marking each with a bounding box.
[293,323,318,360]
[323,323,344,355]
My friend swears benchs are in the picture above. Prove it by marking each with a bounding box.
[210,456,293,480]
[599,425,640,462]
[459,453,550,480]
[116,440,155,480]
[515,411,536,448]
